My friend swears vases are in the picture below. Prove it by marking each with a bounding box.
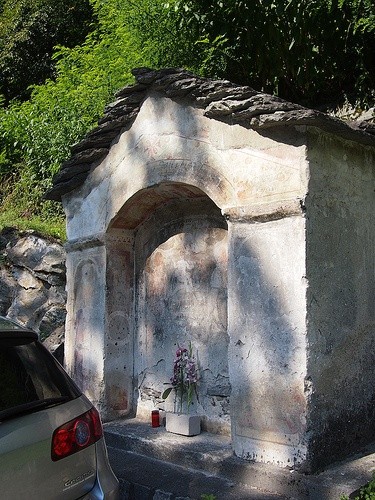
[165,414,200,437]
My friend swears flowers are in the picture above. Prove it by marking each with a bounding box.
[161,341,199,414]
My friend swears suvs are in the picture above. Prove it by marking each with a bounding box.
[0,316,119,500]
